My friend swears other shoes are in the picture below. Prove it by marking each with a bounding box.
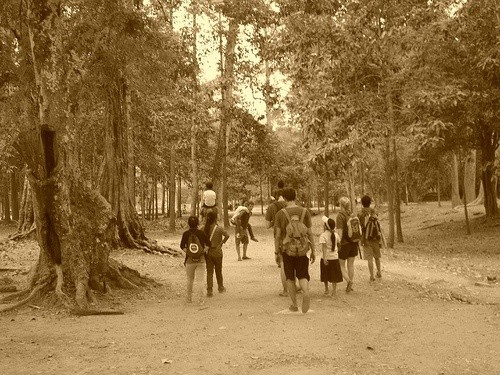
[331,294,337,299]
[207,294,213,298]
[302,295,310,313]
[237,233,241,239]
[238,258,242,261]
[323,293,329,296]
[289,305,298,312]
[369,278,375,283]
[346,281,353,292]
[375,273,382,278]
[279,292,288,297]
[296,289,303,294]
[251,236,258,242]
[199,306,208,310]
[186,298,192,303]
[242,256,251,259]
[219,287,226,293]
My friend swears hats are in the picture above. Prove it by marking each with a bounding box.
[322,216,330,230]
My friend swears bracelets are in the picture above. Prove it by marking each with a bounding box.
[275,252,278,254]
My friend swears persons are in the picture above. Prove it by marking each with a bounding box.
[180,215,212,305]
[200,207,218,224]
[200,212,229,295]
[336,197,359,291]
[275,188,316,314]
[357,196,381,281]
[201,182,214,210]
[235,202,254,261]
[231,200,258,242]
[265,188,303,297]
[321,215,343,296]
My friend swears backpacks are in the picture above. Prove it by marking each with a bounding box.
[281,208,308,257]
[362,208,380,243]
[186,229,204,259]
[339,211,360,242]
